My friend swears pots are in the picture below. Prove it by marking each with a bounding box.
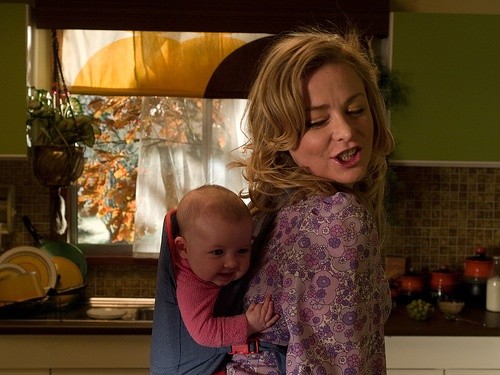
[26,285,89,316]
[23,216,87,282]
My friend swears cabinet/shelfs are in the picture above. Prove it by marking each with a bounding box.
[385,335,500,375]
[379,11,500,168]
[0,2,53,159]
[0,333,151,375]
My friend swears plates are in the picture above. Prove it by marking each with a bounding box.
[0,264,26,280]
[87,309,124,318]
[0,246,56,295]
[49,257,83,289]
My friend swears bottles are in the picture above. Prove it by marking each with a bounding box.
[464,246,493,309]
[487,257,500,312]
[432,266,456,309]
[402,268,422,304]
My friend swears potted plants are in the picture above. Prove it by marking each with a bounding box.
[25,85,101,186]
